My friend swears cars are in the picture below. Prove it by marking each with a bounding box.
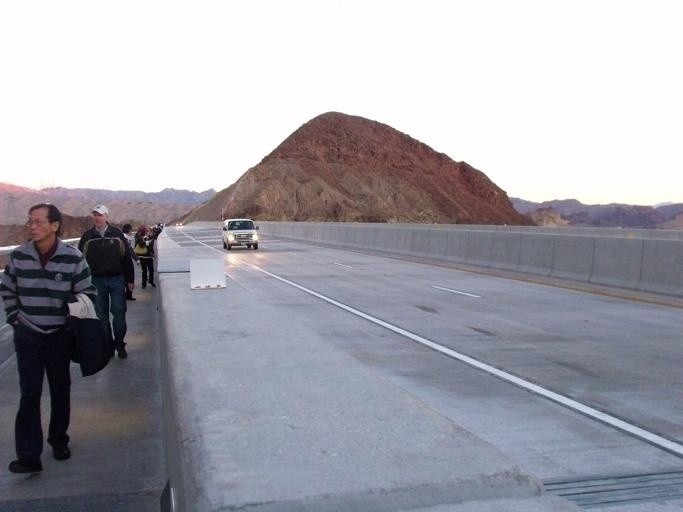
[221,219,260,250]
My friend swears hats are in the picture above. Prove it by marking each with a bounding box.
[91,204,109,215]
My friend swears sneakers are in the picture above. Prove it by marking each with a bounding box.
[125,293,136,301]
[50,442,71,461]
[142,280,156,289]
[116,339,128,359]
[8,458,45,473]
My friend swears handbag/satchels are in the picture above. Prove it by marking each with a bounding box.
[133,243,149,255]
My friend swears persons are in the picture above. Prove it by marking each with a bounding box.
[119,223,136,300]
[0,201,98,473]
[147,221,163,258]
[77,204,135,358]
[132,225,155,289]
